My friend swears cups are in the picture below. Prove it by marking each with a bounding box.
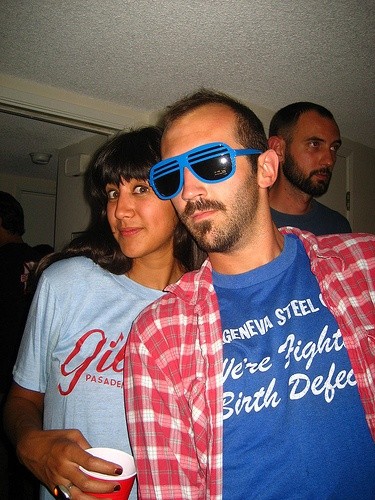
[78,447,137,500]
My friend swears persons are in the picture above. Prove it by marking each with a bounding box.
[122,86,375,500]
[0,124,193,500]
[265,101,352,235]
[0,190,60,500]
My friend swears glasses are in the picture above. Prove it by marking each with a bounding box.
[147,141,263,201]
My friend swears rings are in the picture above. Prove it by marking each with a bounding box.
[53,483,73,500]
